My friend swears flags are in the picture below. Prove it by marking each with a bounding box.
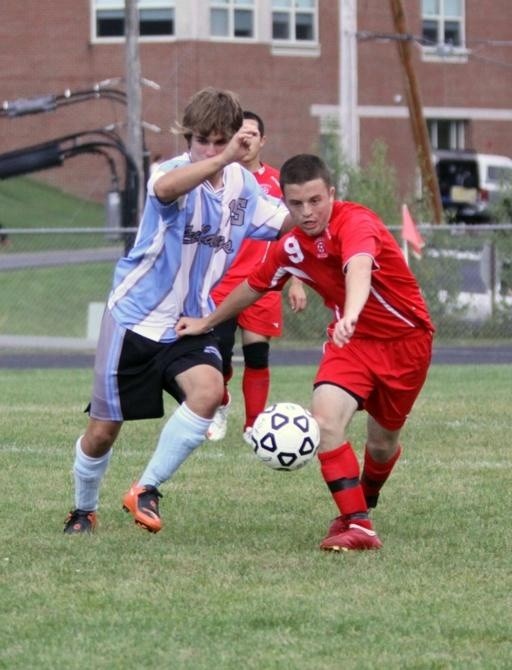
[403,206,426,261]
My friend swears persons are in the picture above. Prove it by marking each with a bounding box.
[207,109,306,448]
[61,86,296,536]
[173,155,434,555]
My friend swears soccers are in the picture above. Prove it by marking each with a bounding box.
[253,403,320,471]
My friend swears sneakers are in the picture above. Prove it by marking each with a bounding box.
[243,427,253,446]
[207,392,231,440]
[320,516,381,551]
[122,480,163,534]
[63,509,96,534]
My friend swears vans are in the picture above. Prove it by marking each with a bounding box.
[416,148,512,220]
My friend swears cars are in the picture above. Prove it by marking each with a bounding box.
[399,247,512,337]
